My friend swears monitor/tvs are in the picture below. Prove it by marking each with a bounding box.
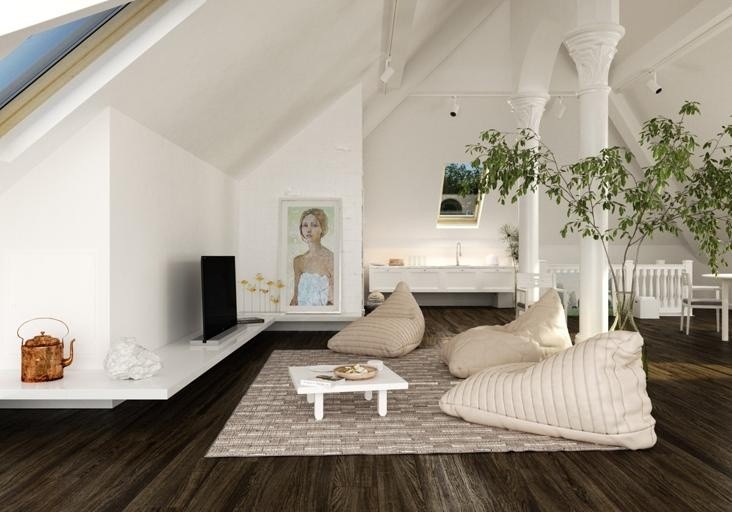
[199,253,237,341]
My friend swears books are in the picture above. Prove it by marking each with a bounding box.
[303,372,345,388]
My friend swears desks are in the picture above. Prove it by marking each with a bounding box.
[702,273,732,341]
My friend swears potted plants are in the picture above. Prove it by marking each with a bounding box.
[466,99,732,388]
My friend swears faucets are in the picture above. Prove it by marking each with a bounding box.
[456,242,461,266]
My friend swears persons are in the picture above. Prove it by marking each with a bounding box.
[290,204,336,308]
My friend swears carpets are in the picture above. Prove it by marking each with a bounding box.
[204,349,625,458]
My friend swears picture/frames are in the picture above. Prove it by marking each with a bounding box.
[279,197,342,315]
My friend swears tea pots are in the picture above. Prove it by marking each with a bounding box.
[19,320,76,383]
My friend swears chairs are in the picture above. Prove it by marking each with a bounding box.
[679,269,732,336]
[438,330,658,450]
[440,289,573,380]
[328,281,426,358]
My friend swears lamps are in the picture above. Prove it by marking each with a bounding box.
[380,58,395,83]
[449,97,459,118]
[647,70,663,95]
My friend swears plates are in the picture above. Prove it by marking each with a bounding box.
[334,366,378,379]
[389,259,404,266]
